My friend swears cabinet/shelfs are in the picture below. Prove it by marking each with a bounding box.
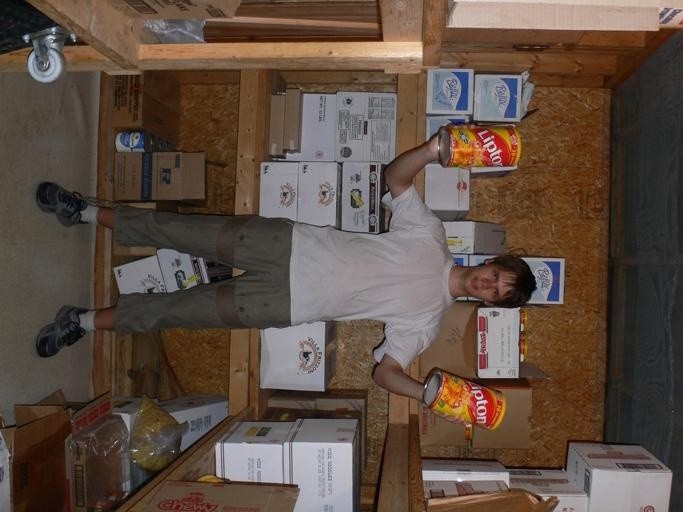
[0,0,683,512]
